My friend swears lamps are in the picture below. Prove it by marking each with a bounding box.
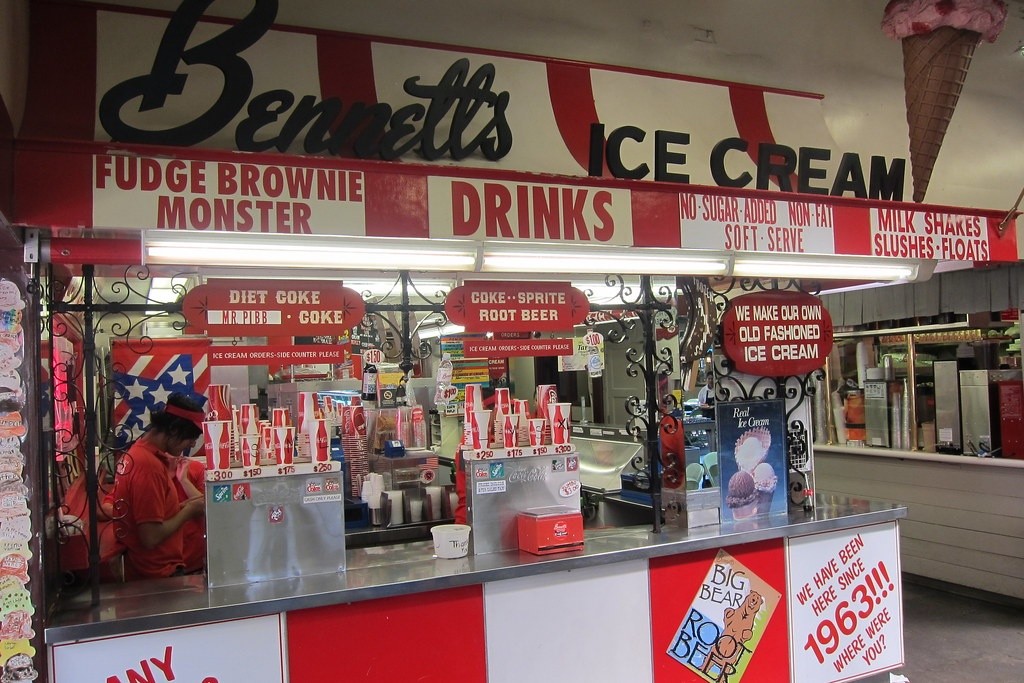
[135,229,937,281]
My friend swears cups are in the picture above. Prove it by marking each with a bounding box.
[811,370,848,445]
[462,384,571,450]
[884,354,912,450]
[208,384,232,421]
[201,392,443,525]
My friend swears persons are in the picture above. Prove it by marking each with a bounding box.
[698,372,717,433]
[98,393,205,578]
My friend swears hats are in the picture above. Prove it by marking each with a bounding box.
[162,404,207,432]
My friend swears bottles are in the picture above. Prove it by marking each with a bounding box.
[434,353,453,405]
[362,363,377,401]
[396,369,417,406]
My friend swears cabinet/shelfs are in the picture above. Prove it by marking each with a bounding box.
[430,412,458,447]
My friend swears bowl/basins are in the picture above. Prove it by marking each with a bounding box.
[430,525,471,559]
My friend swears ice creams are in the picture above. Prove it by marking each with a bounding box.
[726,427,777,521]
